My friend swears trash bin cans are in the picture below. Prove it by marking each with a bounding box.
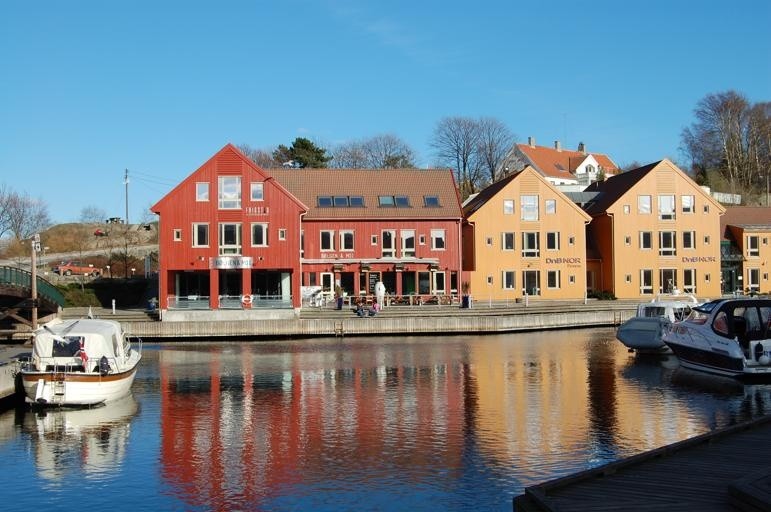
[147,302,156,310]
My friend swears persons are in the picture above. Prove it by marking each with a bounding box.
[357,300,367,317]
[369,299,380,317]
[336,283,343,310]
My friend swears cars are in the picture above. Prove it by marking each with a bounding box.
[53,260,100,276]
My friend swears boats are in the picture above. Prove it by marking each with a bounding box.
[22,319,143,407]
[616,294,769,381]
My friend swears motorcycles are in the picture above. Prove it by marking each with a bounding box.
[353,297,376,315]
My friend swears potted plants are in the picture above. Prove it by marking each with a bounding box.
[335,285,343,309]
[461,280,471,308]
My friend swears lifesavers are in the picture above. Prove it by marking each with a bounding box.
[242,294,252,305]
